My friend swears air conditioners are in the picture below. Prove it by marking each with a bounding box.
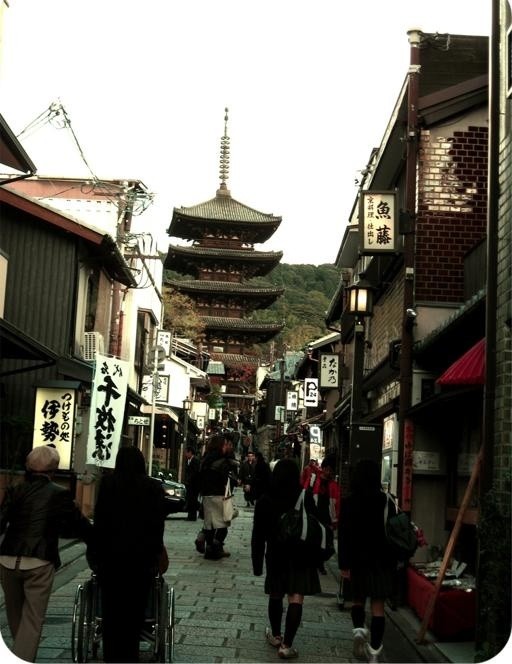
[83,331,104,359]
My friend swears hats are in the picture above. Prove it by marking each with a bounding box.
[26,444,60,472]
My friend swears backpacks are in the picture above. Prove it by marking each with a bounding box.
[383,493,418,557]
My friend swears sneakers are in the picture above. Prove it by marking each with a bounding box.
[365,639,384,662]
[351,628,370,662]
[264,626,283,648]
[196,532,229,559]
[276,644,300,658]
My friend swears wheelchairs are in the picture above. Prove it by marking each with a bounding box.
[72,572,174,664]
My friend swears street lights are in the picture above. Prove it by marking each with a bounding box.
[181,397,192,483]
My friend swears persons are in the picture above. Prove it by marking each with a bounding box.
[89,446,167,661]
[1,446,93,662]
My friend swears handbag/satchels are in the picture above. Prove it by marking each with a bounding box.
[222,477,240,523]
[279,488,331,549]
